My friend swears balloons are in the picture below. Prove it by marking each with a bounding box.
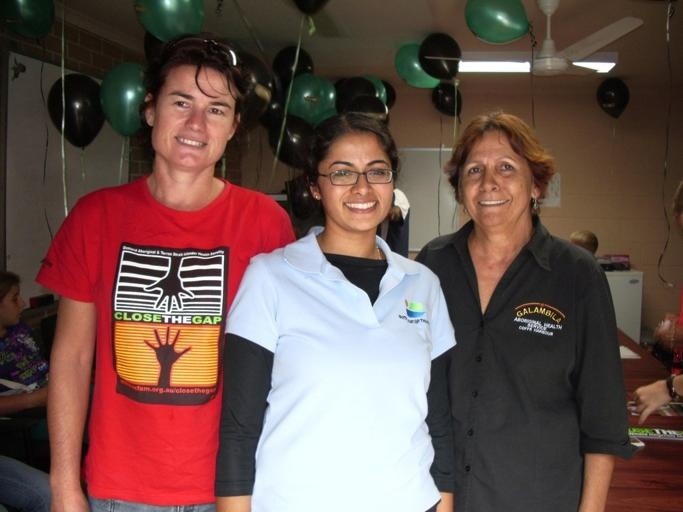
[144,31,170,68]
[47,73,104,148]
[465,0,533,47]
[235,44,396,169]
[416,32,461,81]
[133,1,208,44]
[432,82,463,117]
[97,62,151,137]
[596,77,631,119]
[395,31,442,88]
[6,0,55,40]
[292,0,326,17]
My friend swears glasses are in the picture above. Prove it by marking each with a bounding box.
[320,168,395,187]
[172,38,238,67]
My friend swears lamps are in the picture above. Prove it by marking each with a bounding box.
[441,50,620,76]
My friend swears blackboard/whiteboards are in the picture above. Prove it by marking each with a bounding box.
[0,51,132,327]
[395,148,456,252]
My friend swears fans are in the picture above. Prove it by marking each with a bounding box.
[422,1,643,79]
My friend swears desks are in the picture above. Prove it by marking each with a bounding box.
[598,326,682,510]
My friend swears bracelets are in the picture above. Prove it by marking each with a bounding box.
[667,374,682,403]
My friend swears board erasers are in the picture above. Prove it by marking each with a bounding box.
[30,293,54,309]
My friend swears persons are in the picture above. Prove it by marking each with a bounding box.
[374,186,411,261]
[214,110,458,511]
[570,227,599,256]
[0,269,49,511]
[412,111,637,512]
[631,372,682,427]
[35,34,297,512]
